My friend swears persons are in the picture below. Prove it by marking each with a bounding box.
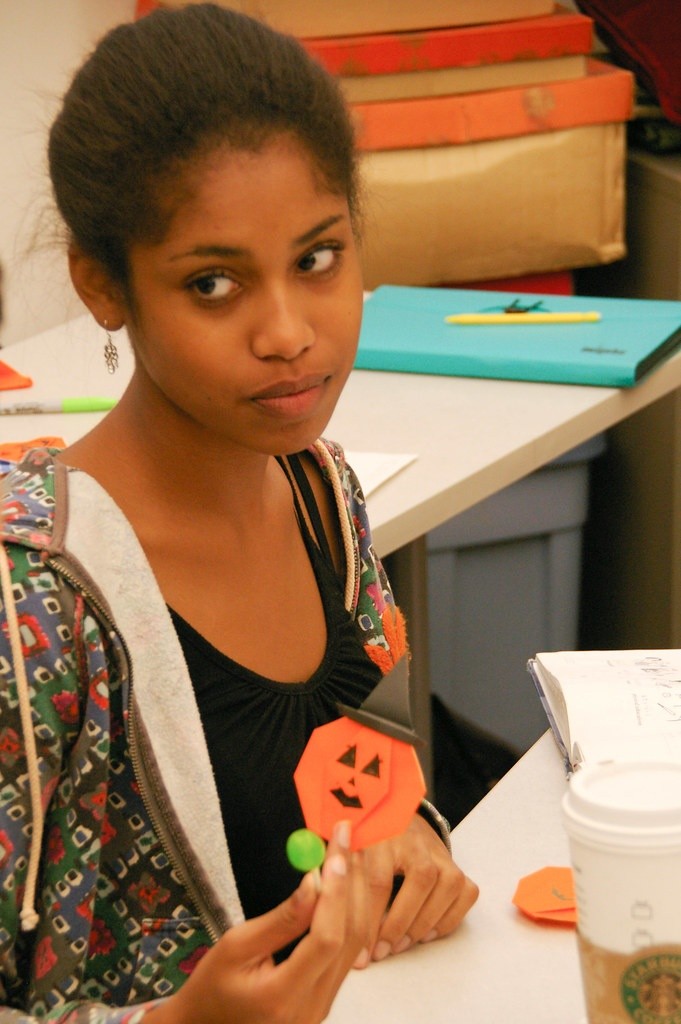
[0,4,481,1024]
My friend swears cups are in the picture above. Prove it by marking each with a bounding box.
[562,758,681,1024]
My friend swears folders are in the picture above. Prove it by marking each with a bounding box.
[351,282,681,387]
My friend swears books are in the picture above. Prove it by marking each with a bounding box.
[349,284,681,388]
[528,650,681,781]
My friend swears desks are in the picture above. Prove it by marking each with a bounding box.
[0,305,679,799]
[328,721,587,1024]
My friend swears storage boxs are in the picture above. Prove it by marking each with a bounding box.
[321,49,636,284]
[165,0,556,44]
[298,0,590,106]
[428,462,590,752]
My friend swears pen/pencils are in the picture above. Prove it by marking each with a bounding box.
[444,310,600,324]
[0,394,117,413]
